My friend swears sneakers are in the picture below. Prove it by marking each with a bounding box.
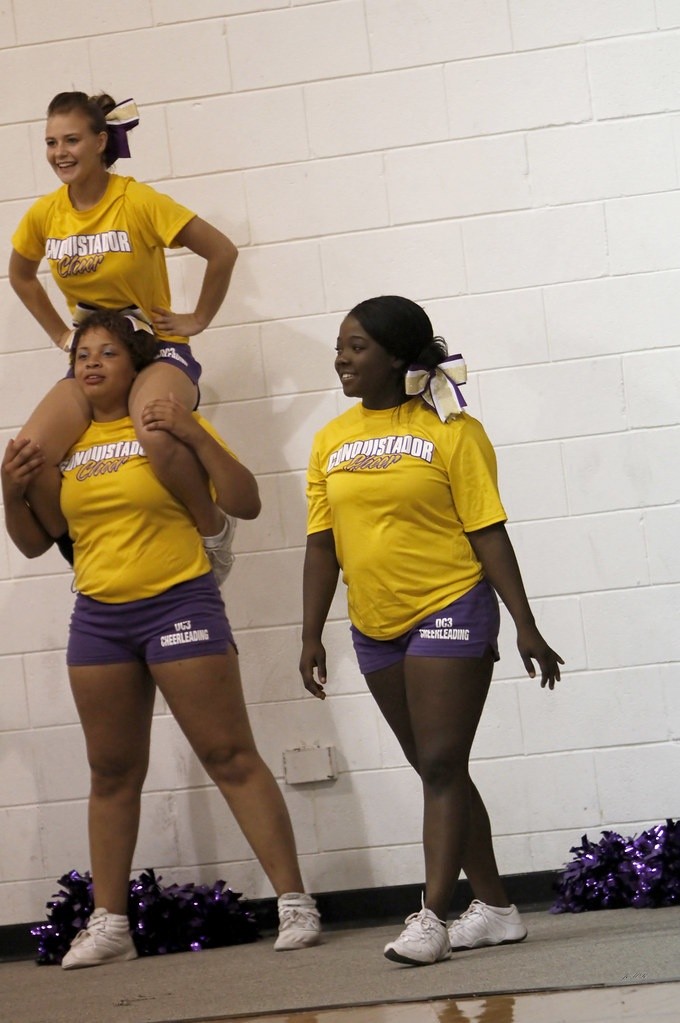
[62,908,138,970]
[447,899,528,952]
[273,892,322,951]
[383,891,452,965]
[193,504,238,588]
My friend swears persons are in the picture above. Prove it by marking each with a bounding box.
[299,296,567,963]
[7,93,238,586]
[0,310,321,967]
